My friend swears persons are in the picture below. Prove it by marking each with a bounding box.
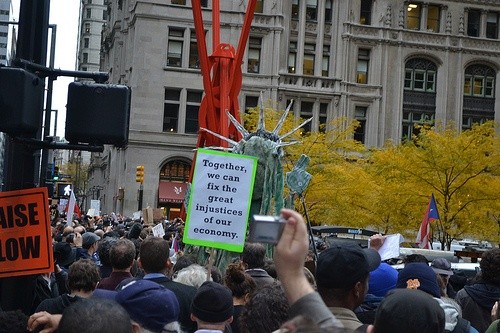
[0,196,500,333]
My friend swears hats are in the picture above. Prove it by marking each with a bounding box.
[82,232,101,246]
[395,263,441,298]
[91,279,180,332]
[53,240,74,266]
[430,258,453,275]
[315,243,382,290]
[369,263,399,297]
[190,281,234,324]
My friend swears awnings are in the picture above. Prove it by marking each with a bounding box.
[159,181,186,203]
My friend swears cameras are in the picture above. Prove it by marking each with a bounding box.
[249,214,289,244]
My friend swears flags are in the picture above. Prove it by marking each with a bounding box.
[66,190,81,225]
[415,193,442,250]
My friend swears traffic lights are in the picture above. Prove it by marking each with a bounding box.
[0,67,44,134]
[56,182,73,197]
[135,165,144,183]
